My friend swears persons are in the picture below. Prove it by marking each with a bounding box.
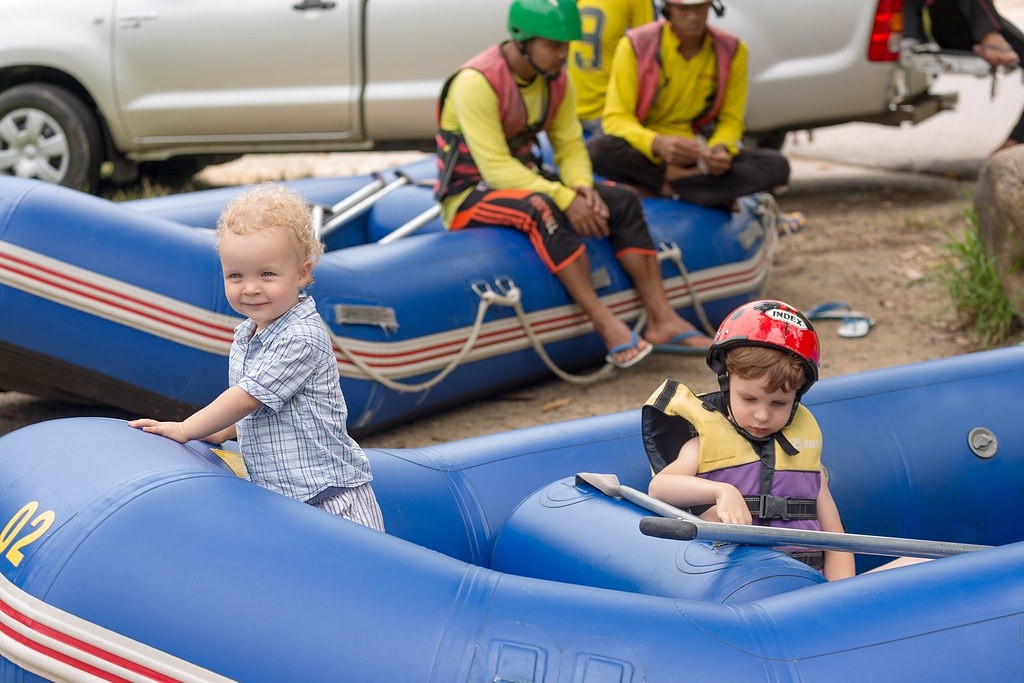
[434,0,714,368]
[587,0,789,212]
[905,0,1024,144]
[129,189,384,532]
[642,299,855,581]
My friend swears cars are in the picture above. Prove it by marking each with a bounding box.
[0,1,960,202]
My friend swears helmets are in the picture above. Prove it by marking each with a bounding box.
[704,298,822,384]
[661,1,725,21]
[506,0,584,44]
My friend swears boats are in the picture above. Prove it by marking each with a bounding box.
[0,173,782,438]
[0,341,1024,683]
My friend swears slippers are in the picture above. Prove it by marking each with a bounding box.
[604,328,714,369]
[806,299,877,339]
[971,41,1022,68]
